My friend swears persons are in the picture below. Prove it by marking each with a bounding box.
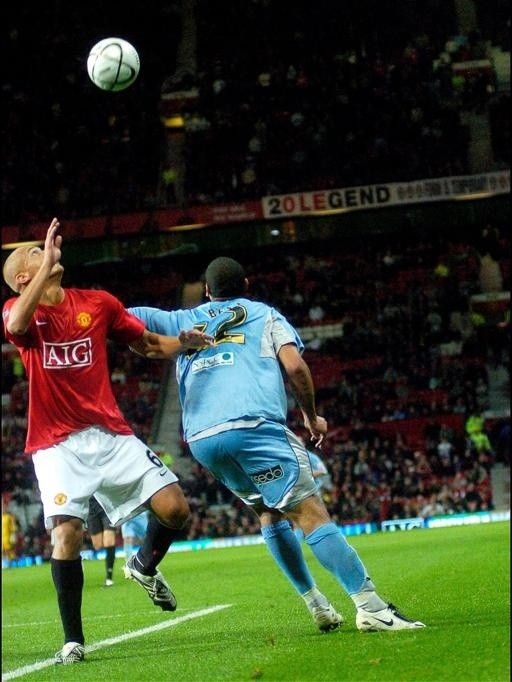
[123,255,428,633]
[3,216,221,665]
[2,228,512,588]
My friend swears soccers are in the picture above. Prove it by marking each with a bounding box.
[87,37,141,91]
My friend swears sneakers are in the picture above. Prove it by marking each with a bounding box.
[56,643,85,664]
[105,578,112,586]
[312,603,343,632]
[127,555,177,611]
[356,604,426,631]
